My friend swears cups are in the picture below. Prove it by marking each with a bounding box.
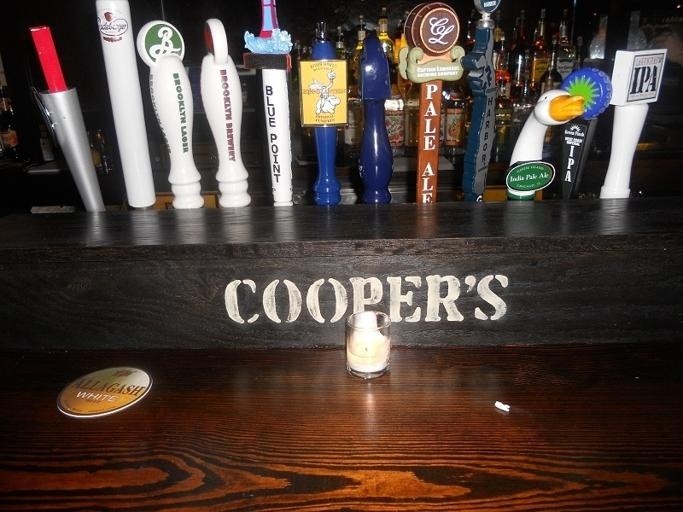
[345,311,391,381]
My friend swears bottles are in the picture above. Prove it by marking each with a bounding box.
[513,80,535,144]
[445,82,466,154]
[496,35,506,70]
[0,88,25,161]
[342,78,362,156]
[336,27,348,59]
[352,15,366,84]
[405,81,419,156]
[495,80,513,163]
[492,10,502,69]
[531,7,550,91]
[551,9,576,81]
[509,10,532,96]
[385,84,406,157]
[377,16,394,83]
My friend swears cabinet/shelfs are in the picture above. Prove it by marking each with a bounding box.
[0,1,683,512]
